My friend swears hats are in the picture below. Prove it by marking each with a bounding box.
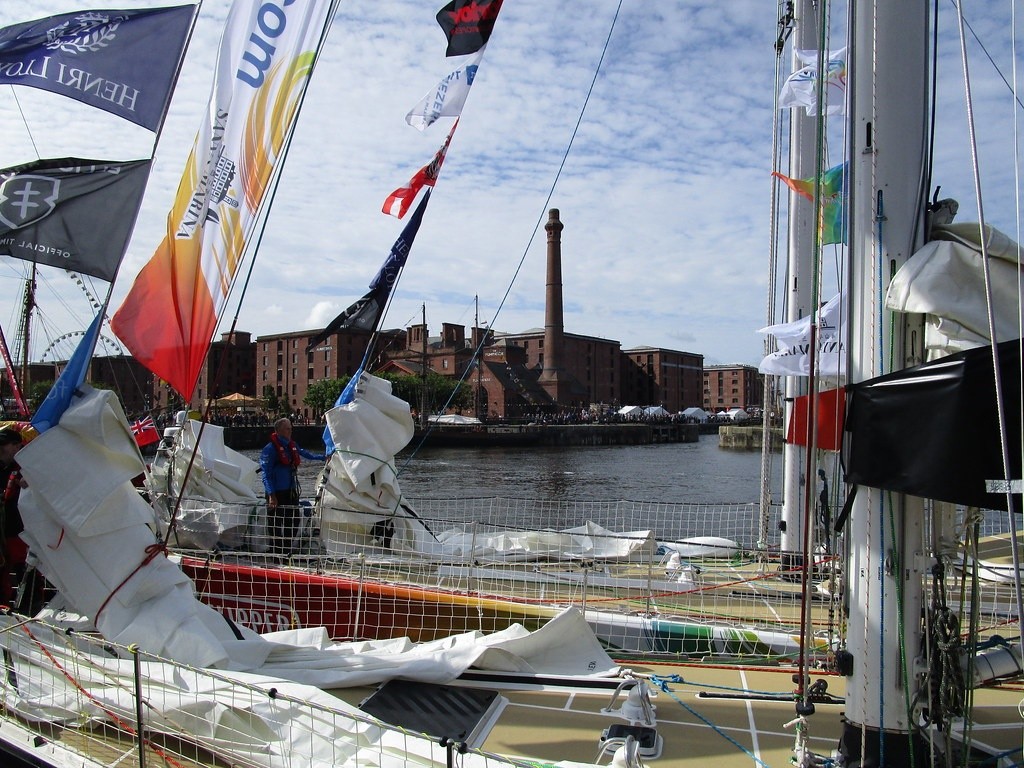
[0,429,22,444]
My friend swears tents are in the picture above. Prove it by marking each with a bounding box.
[618,405,750,423]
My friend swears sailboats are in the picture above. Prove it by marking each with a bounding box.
[1,2,1024,767]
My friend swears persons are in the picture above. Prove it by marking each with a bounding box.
[260,418,331,566]
[0,428,44,687]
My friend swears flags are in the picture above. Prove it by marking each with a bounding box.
[404,43,487,133]
[771,161,849,250]
[381,116,459,220]
[303,187,433,356]
[776,45,849,118]
[0,156,152,284]
[755,287,847,377]
[30,303,105,434]
[1,4,197,133]
[437,0,503,57]
[110,0,342,405]
